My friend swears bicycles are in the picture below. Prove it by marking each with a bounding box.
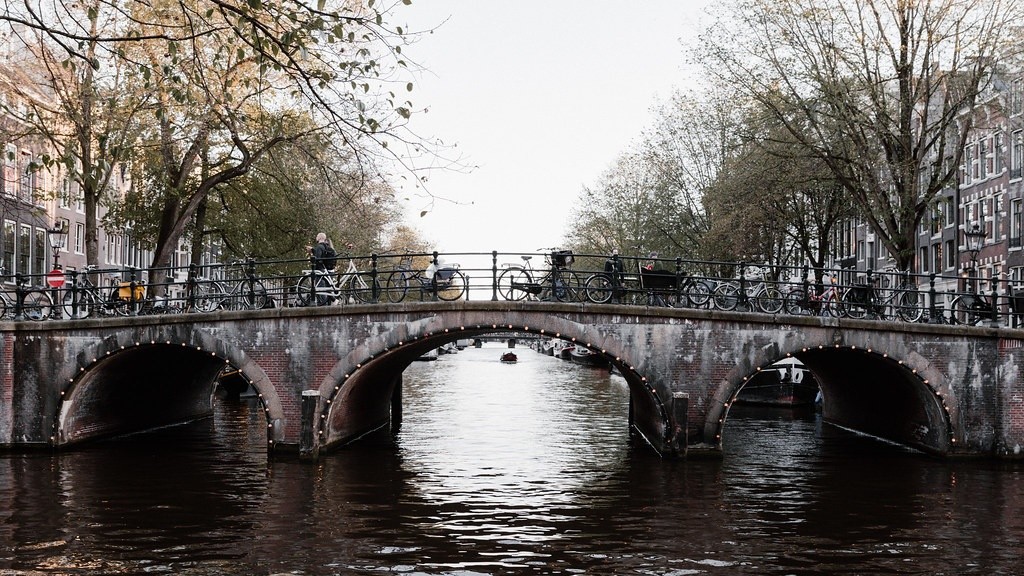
[0,266,53,321]
[950,268,1023,327]
[842,267,926,323]
[387,249,466,302]
[63,264,146,320]
[713,266,784,313]
[584,258,711,315]
[497,247,580,302]
[783,272,851,318]
[297,253,381,307]
[193,259,268,313]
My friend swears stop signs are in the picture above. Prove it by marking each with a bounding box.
[46,270,64,287]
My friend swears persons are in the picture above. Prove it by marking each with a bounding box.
[306,231,333,306]
[649,251,664,307]
[605,249,625,303]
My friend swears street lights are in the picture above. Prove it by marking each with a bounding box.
[46,220,68,320]
[964,223,989,326]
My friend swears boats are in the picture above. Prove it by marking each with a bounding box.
[439,339,475,354]
[518,340,622,376]
[415,347,438,362]
[500,352,517,364]
[728,362,819,411]
[458,346,464,350]
[448,349,458,354]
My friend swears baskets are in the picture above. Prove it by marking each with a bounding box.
[555,250,575,266]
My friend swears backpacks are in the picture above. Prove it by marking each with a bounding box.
[320,243,337,270]
